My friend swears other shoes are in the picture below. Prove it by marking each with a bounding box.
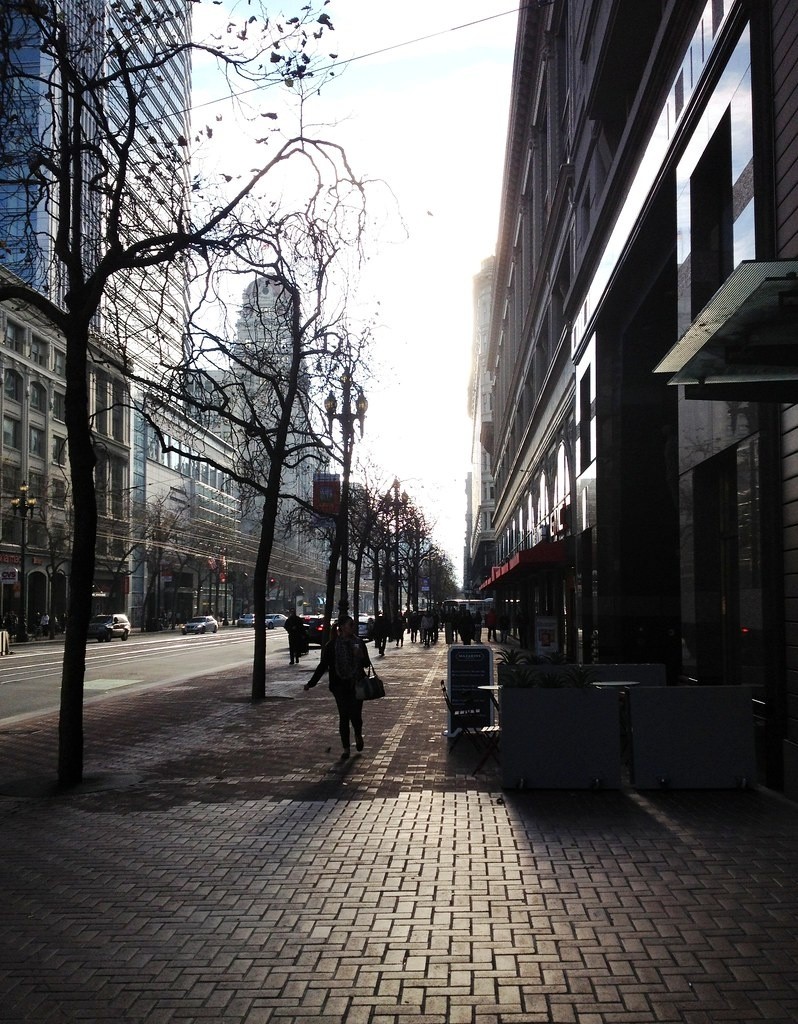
[289,662,294,665]
[356,736,364,751]
[341,751,350,759]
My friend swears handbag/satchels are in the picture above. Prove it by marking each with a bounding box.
[355,661,385,700]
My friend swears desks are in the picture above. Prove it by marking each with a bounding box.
[473,681,639,774]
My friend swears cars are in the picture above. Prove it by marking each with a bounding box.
[237,614,255,628]
[347,612,383,642]
[265,614,288,629]
[181,615,219,635]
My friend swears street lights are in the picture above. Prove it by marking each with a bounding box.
[9,480,38,644]
[325,365,369,631]
[382,477,408,620]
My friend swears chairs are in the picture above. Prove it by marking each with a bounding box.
[471,725,502,777]
[626,680,759,789]
[440,679,491,754]
[498,687,623,791]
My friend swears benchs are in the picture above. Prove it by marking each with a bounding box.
[498,663,666,688]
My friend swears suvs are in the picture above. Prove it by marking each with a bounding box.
[299,613,325,647]
[86,614,131,643]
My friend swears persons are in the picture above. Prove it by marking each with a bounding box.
[365,605,511,656]
[303,614,371,760]
[150,607,226,631]
[283,608,304,664]
[1,606,67,641]
